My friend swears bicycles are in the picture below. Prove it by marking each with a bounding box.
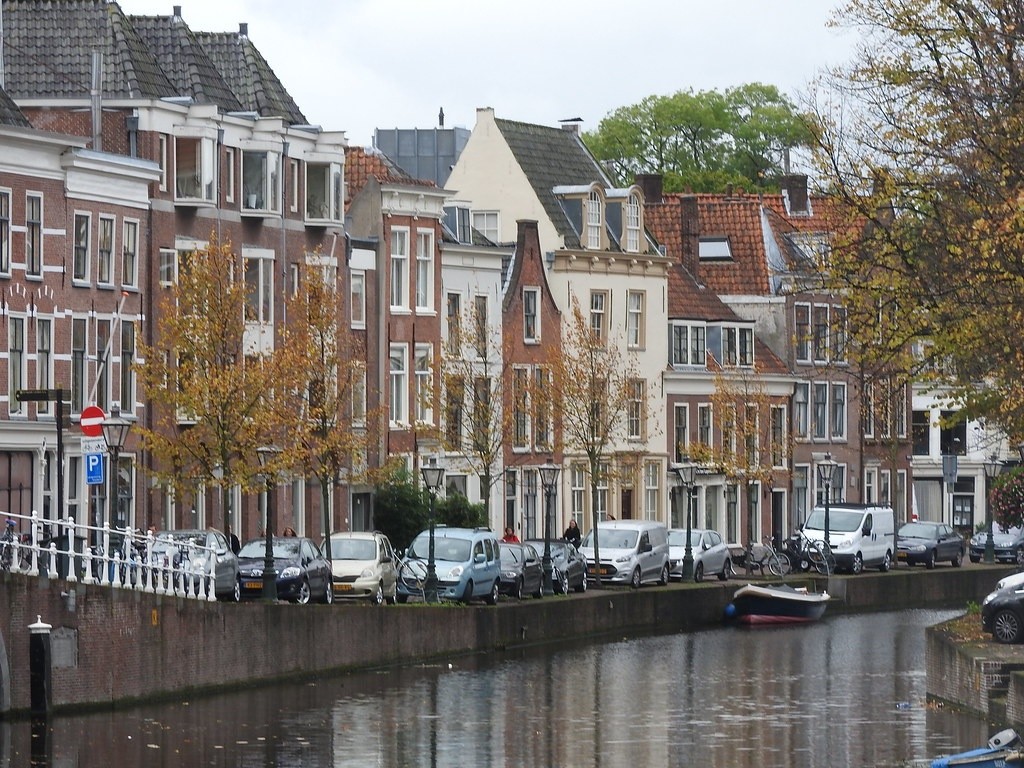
[730,529,836,577]
[0,516,192,597]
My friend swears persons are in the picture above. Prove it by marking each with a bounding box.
[283,526,297,537]
[230,532,240,554]
[504,527,520,544]
[608,514,616,520]
[564,519,582,550]
[149,524,158,533]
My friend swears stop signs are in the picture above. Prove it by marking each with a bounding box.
[80,406,106,436]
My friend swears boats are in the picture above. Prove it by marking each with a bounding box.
[930,728,1024,768]
[734,584,831,627]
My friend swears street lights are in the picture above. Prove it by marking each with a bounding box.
[256,443,279,601]
[674,462,696,582]
[818,451,839,550]
[422,462,443,604]
[983,453,1005,565]
[537,457,556,597]
[101,403,132,561]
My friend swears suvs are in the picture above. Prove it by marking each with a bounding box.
[145,526,243,603]
[319,529,399,606]
[396,524,502,605]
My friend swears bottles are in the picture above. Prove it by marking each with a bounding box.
[896,702,909,709]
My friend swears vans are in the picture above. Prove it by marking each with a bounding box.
[803,501,895,575]
[579,518,671,589]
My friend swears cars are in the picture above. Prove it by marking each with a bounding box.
[981,572,1024,644]
[968,519,1024,567]
[668,529,732,581]
[237,537,332,604]
[499,538,588,601]
[897,520,967,570]
